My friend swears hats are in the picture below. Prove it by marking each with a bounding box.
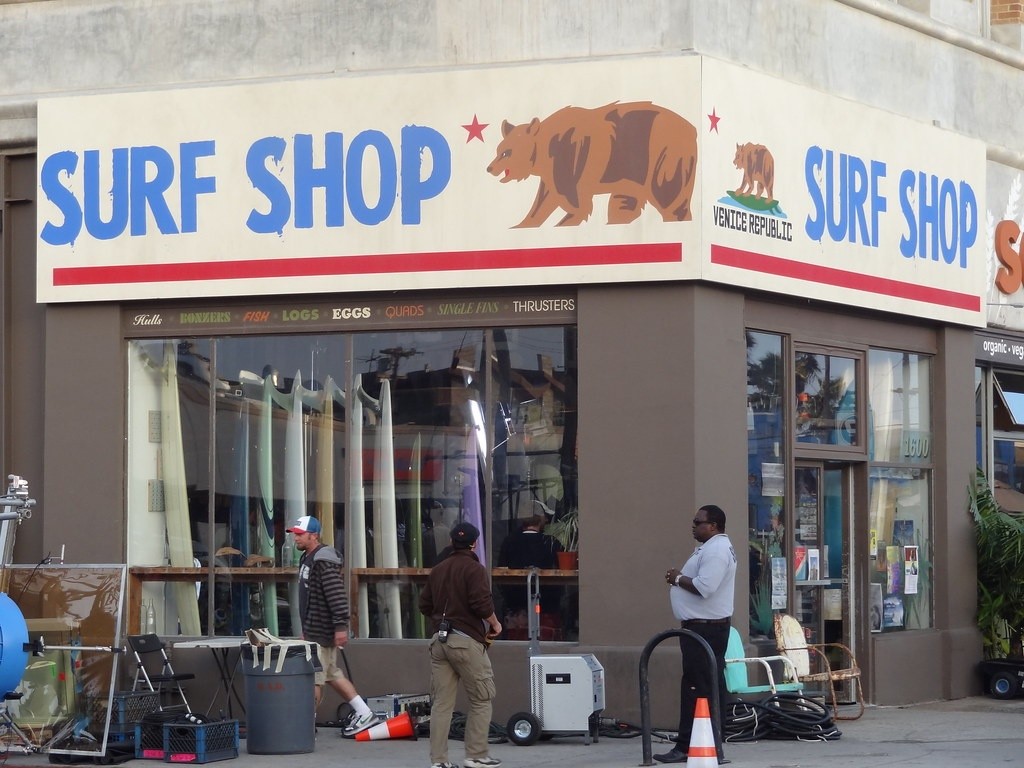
[532,500,555,514]
[285,516,321,533]
[450,523,481,549]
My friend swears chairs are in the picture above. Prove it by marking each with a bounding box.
[723,626,808,711]
[128,634,196,716]
[772,613,866,723]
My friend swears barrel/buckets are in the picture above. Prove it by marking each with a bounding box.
[240,644,317,754]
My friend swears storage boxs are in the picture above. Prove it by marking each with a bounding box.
[88,690,239,764]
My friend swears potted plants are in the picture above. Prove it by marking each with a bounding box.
[544,510,579,570]
[968,460,1024,699]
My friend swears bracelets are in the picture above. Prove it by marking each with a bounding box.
[675,574,683,586]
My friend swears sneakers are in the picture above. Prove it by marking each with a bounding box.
[343,712,380,736]
[464,755,502,768]
[431,761,459,768]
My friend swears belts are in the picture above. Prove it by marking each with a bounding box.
[681,618,731,627]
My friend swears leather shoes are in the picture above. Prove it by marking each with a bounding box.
[653,749,688,763]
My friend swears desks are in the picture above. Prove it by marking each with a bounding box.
[172,639,249,721]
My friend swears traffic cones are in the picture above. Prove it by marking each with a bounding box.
[684,697,721,768]
[354,710,418,741]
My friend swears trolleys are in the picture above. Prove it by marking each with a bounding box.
[507,572,620,745]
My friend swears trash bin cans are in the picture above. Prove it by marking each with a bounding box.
[240,641,323,755]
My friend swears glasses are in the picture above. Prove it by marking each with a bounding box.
[692,518,713,526]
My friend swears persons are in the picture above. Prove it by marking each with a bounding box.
[419,522,502,768]
[286,516,379,735]
[653,504,738,762]
[498,507,569,614]
